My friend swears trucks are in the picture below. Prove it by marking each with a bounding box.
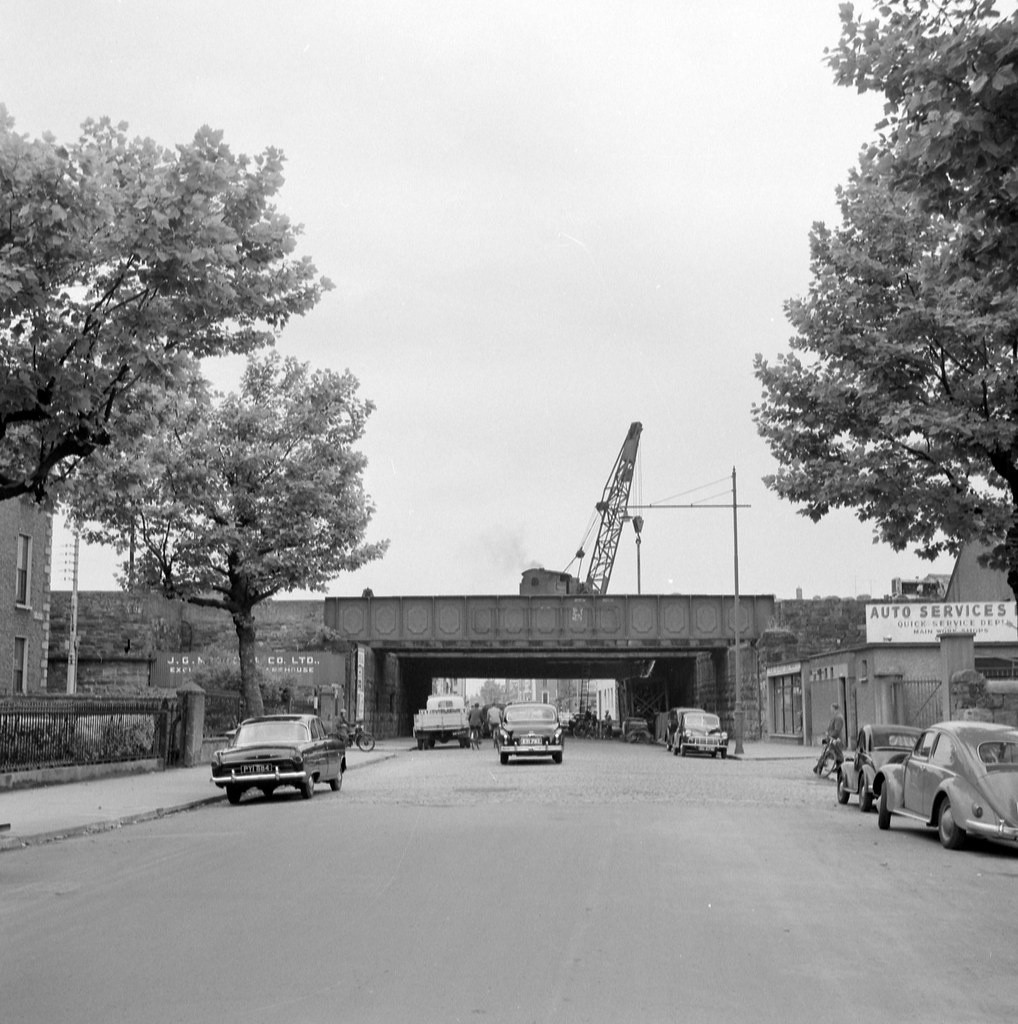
[412,694,470,749]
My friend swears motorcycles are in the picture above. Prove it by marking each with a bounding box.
[327,724,377,753]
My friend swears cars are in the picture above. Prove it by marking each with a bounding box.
[836,723,924,812]
[671,712,729,759]
[872,721,1018,848]
[664,706,706,750]
[208,713,348,800]
[495,702,565,765]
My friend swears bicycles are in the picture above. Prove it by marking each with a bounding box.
[469,724,482,750]
[816,734,840,779]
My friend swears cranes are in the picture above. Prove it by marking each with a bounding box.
[565,421,646,595]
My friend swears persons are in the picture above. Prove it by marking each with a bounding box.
[827,701,844,776]
[337,709,355,745]
[486,702,503,740]
[469,703,482,744]
[584,706,613,739]
[653,707,661,721]
[635,706,642,718]
